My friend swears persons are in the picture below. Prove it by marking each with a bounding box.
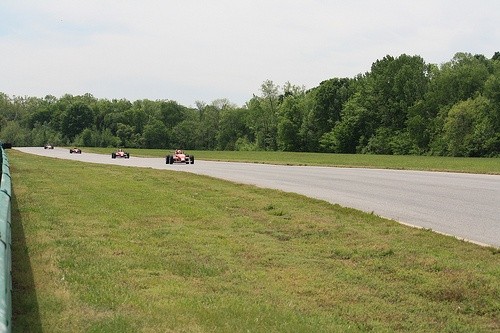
[120,149,122,152]
[74,147,77,149]
[177,150,182,154]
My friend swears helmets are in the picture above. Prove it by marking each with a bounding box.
[177,150,182,154]
